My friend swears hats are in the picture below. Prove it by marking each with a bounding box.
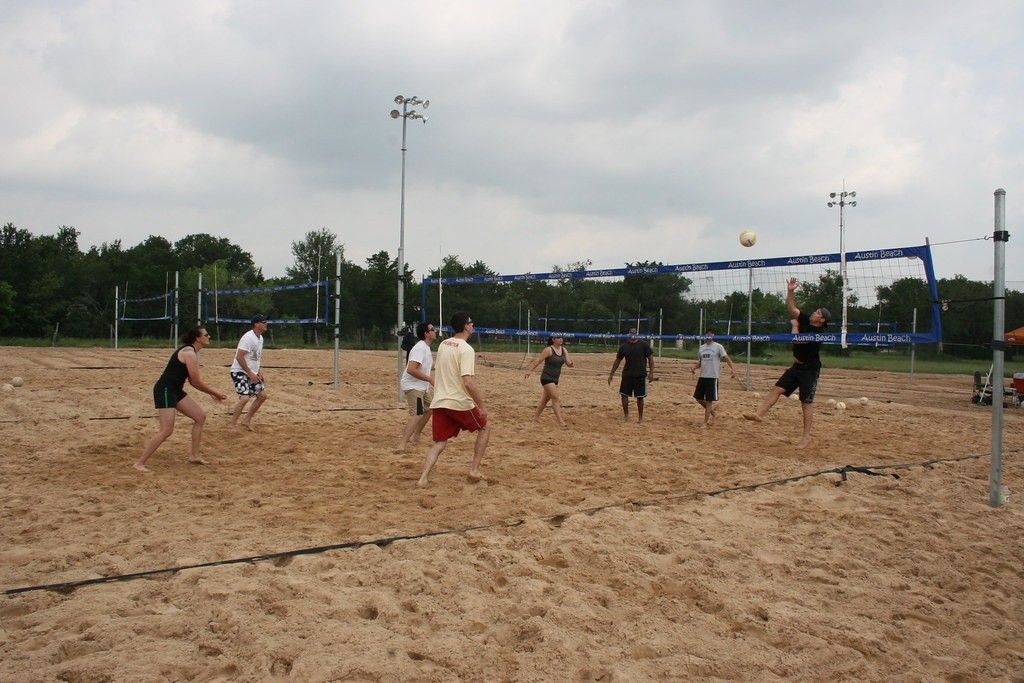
[704,328,714,335]
[251,315,265,323]
[820,307,831,329]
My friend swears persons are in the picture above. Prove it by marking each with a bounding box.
[524,336,574,429]
[418,312,490,483]
[399,322,436,449]
[742,278,832,450]
[133,326,228,472]
[607,326,655,419]
[230,314,269,431]
[691,329,737,422]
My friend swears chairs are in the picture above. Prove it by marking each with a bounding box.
[973,371,993,398]
[1013,373,1024,408]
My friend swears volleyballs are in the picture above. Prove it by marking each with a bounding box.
[739,229,757,248]
[3,376,24,392]
[790,393,869,411]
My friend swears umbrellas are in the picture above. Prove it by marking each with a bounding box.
[1004,326,1024,345]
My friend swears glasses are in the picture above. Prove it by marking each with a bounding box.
[628,331,638,334]
[553,335,564,339]
[427,327,435,332]
